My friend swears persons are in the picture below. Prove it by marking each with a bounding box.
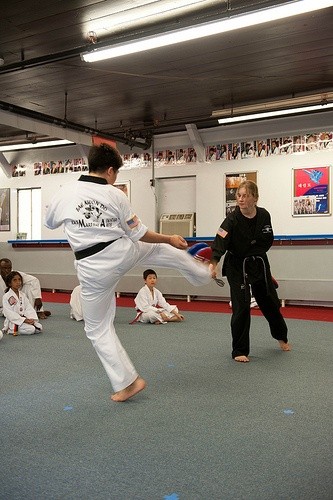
[70,282,86,321]
[209,181,290,363]
[44,142,212,403]
[0,258,52,319]
[3,271,43,336]
[0,330,3,341]
[12,132,333,177]
[134,269,185,325]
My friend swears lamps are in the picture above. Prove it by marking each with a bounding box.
[77,0,333,64]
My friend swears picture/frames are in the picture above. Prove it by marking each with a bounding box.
[290,165,332,218]
[113,179,131,205]
[222,170,259,222]
